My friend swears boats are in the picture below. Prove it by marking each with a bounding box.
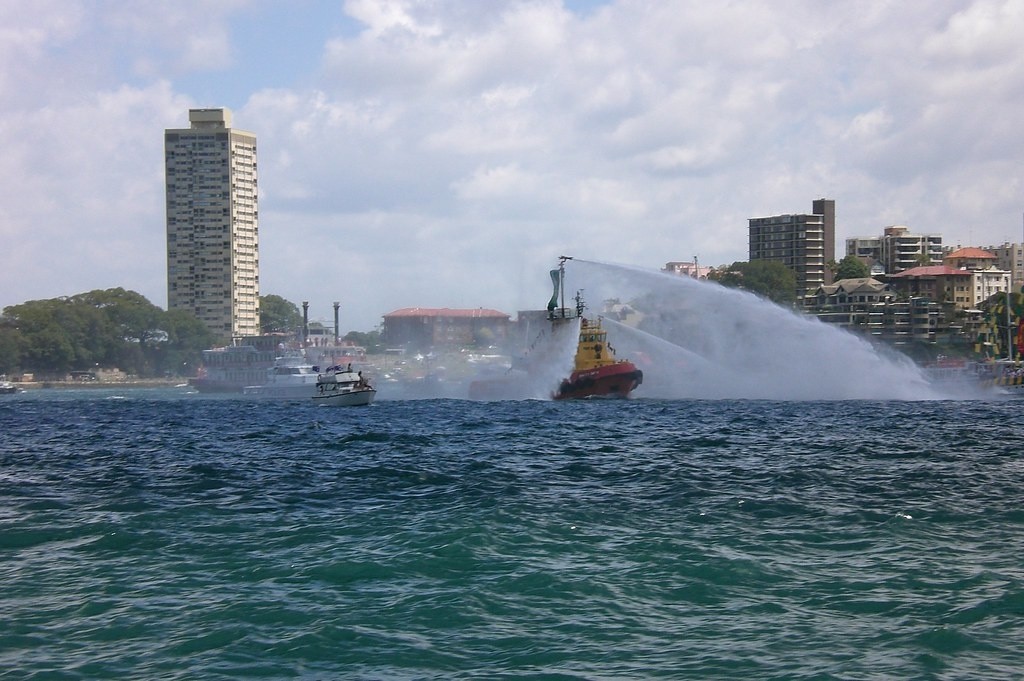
[187,298,377,394]
[465,255,644,399]
[0,374,17,393]
[243,356,377,406]
